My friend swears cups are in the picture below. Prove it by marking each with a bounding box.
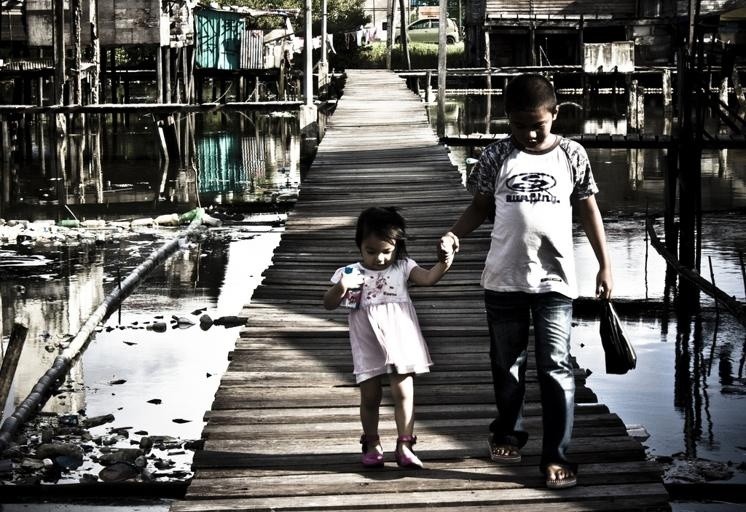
[339,268,365,310]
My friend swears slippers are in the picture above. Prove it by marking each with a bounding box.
[543,462,579,491]
[485,432,523,465]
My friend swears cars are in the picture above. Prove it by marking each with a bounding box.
[395,17,459,44]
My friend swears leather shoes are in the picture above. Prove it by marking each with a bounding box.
[393,434,425,471]
[358,432,384,467]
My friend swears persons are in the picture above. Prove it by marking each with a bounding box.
[436,75,615,489]
[323,205,456,473]
